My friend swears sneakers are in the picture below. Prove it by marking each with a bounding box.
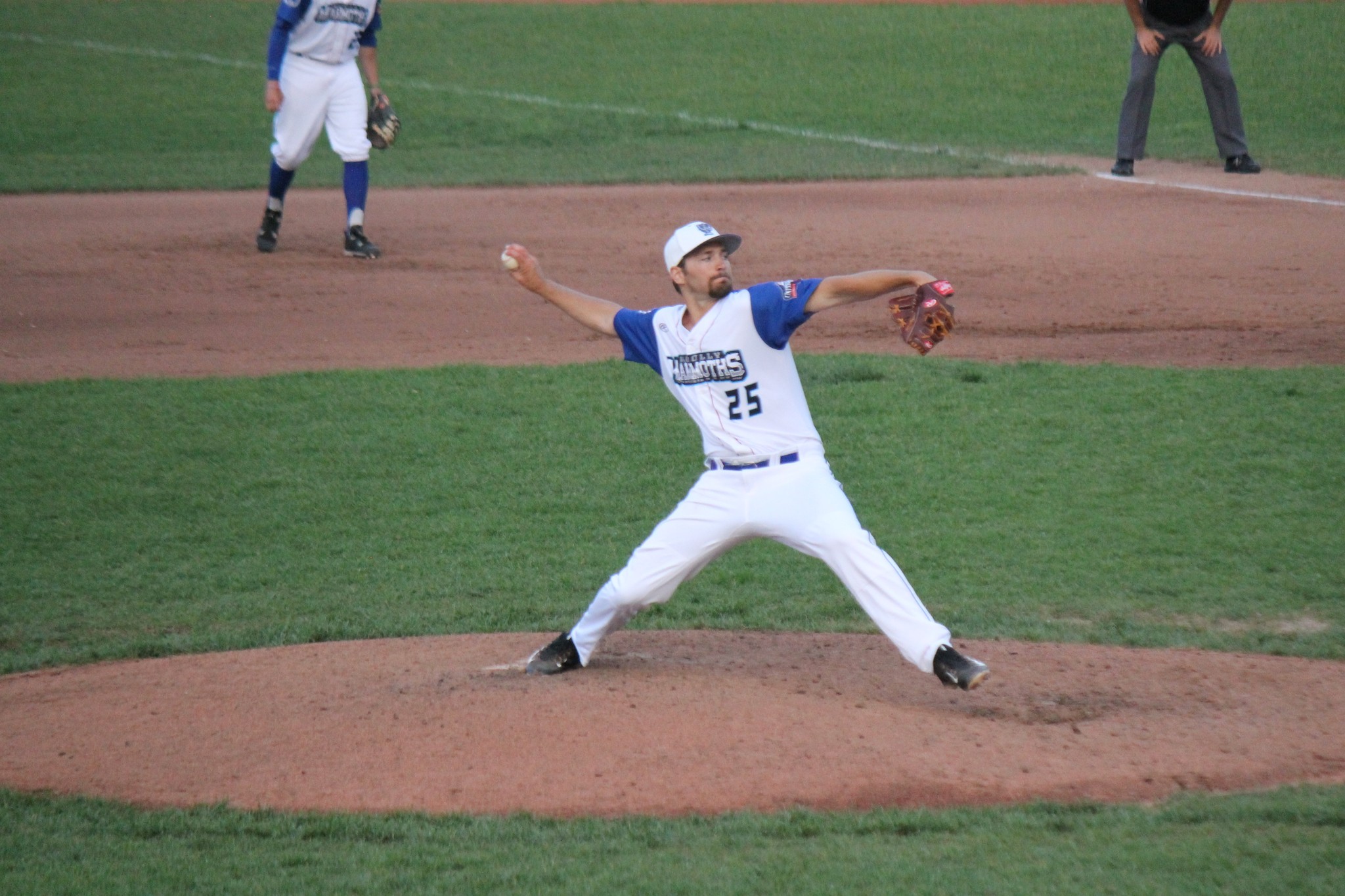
[1111,158,1134,175]
[257,204,282,254]
[933,646,991,691]
[1224,154,1260,173]
[342,224,380,258]
[526,633,579,675]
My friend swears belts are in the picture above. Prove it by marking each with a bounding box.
[712,452,798,471]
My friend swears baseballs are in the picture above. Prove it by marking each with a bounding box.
[501,250,520,271]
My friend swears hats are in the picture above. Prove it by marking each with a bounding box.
[664,222,741,273]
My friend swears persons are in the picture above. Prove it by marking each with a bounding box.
[1111,1,1261,177]
[253,1,392,258]
[499,221,990,692]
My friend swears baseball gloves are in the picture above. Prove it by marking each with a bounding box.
[889,280,955,357]
[366,97,401,151]
